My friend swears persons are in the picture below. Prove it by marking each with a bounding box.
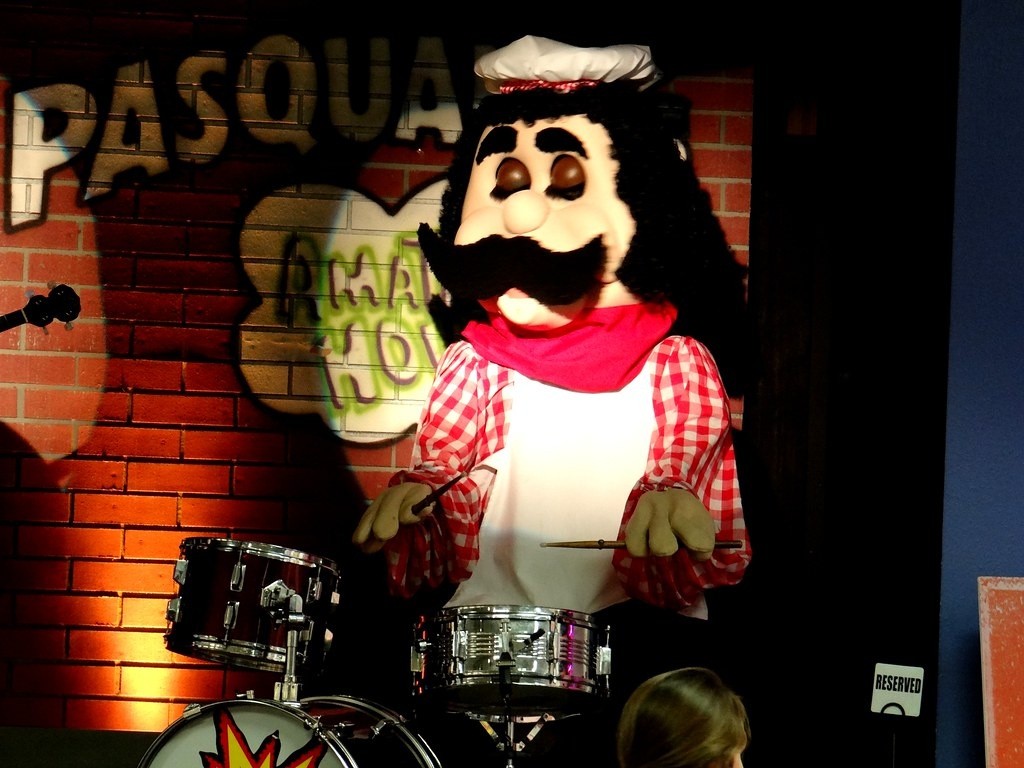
[351,35,752,620]
[617,668,751,768]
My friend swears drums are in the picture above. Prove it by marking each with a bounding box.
[136,695,442,768]
[165,536,342,681]
[410,603,603,718]
